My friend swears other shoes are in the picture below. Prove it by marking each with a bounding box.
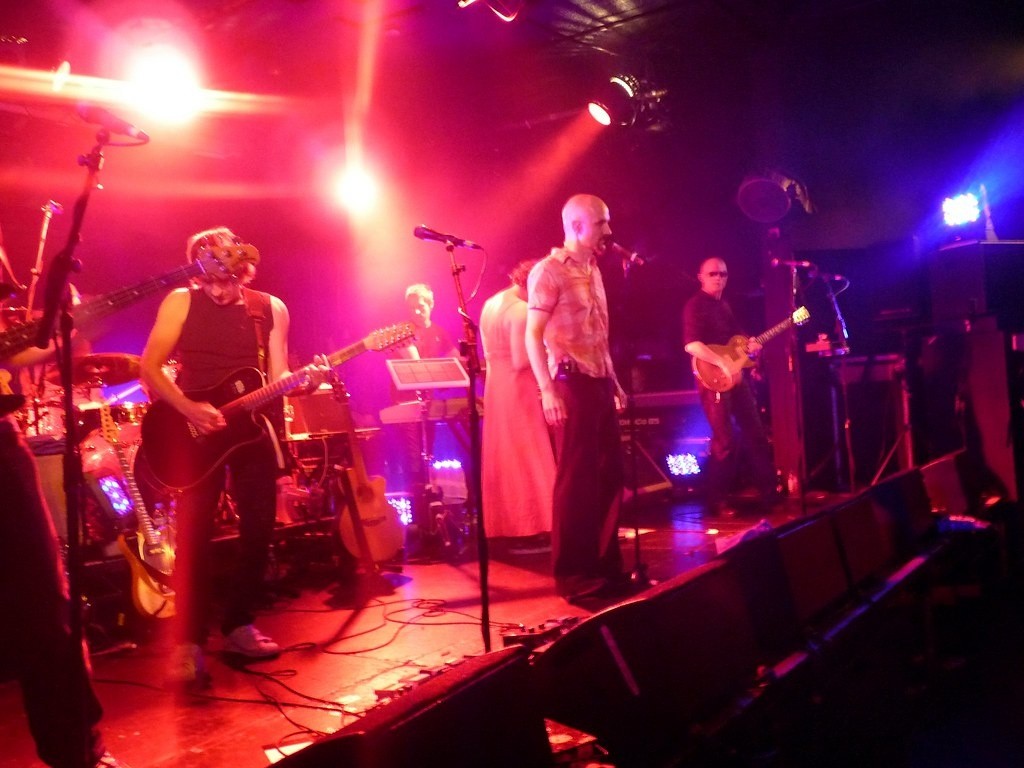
[719,503,736,519]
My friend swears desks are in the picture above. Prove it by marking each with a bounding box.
[828,353,914,491]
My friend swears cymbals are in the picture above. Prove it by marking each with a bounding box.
[9,327,79,368]
[44,352,143,389]
[0,305,44,319]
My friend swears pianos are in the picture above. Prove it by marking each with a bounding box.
[379,398,483,528]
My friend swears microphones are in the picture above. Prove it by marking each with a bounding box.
[77,101,149,141]
[771,258,812,268]
[414,226,483,249]
[607,241,644,265]
[807,270,843,281]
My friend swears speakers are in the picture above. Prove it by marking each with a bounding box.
[919,447,983,516]
[264,645,556,768]
[620,435,672,508]
[534,466,935,750]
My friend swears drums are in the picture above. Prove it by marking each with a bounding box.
[78,422,140,523]
[20,391,66,438]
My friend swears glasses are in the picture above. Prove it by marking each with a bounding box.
[699,271,728,277]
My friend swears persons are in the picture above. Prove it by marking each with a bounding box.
[524,193,629,601]
[0,284,130,768]
[479,261,557,556]
[389,284,471,534]
[683,259,772,519]
[139,228,329,689]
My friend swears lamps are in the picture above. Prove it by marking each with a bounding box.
[589,64,672,132]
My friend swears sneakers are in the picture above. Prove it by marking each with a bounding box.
[221,623,277,657]
[162,645,201,681]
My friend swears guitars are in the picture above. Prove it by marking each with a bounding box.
[0,235,262,421]
[329,374,407,564]
[99,404,176,619]
[689,307,811,392]
[139,323,413,492]
